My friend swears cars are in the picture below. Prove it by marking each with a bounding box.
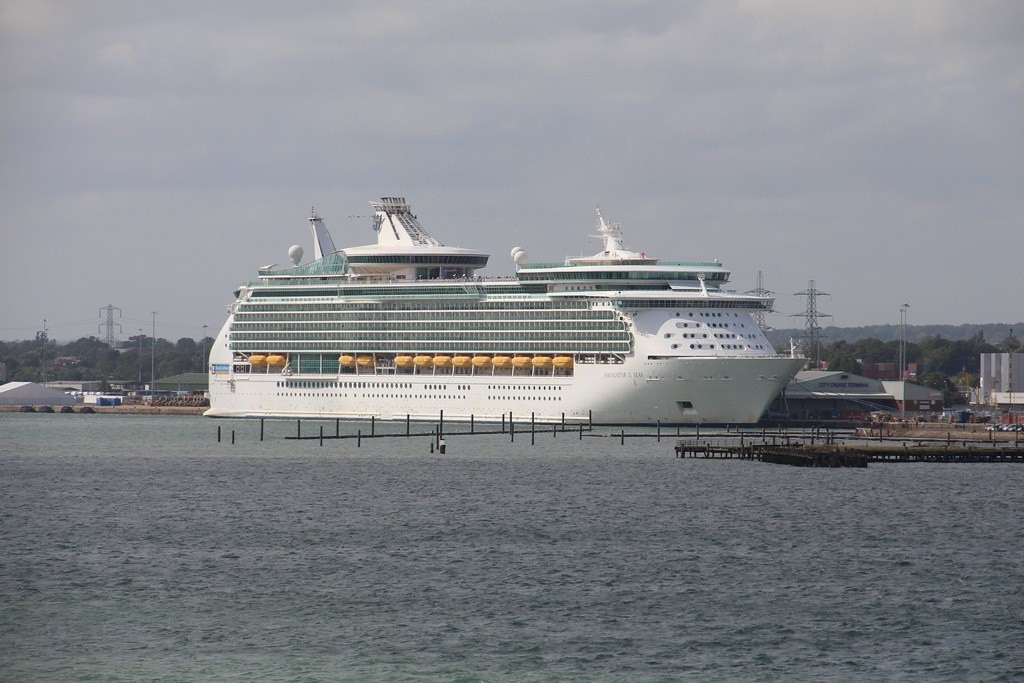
[984,423,1024,432]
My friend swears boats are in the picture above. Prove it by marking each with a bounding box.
[339,354,355,368]
[413,356,432,368]
[512,357,532,369]
[266,354,286,367]
[532,357,551,368]
[452,356,471,368]
[394,356,414,368]
[471,356,491,369]
[552,356,572,368]
[248,354,266,366]
[491,356,511,369]
[433,355,451,367]
[357,355,375,368]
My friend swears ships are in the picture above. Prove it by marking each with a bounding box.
[201,196,808,428]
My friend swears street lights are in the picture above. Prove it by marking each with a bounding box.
[898,303,910,380]
